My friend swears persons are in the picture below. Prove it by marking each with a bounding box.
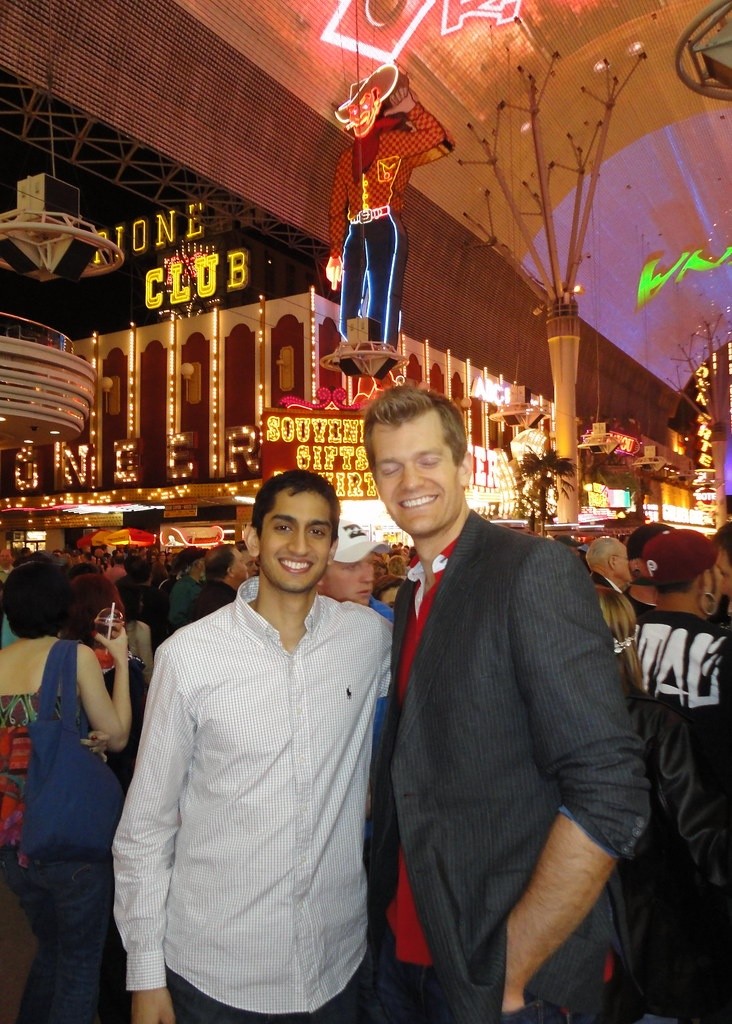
[318,521,417,815]
[112,471,395,1024]
[363,386,648,1024]
[552,523,732,1024]
[0,541,261,1024]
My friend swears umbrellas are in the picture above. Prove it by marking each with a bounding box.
[77,528,159,548]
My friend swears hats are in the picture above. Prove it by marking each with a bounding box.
[331,518,391,563]
[643,529,719,584]
[178,545,205,571]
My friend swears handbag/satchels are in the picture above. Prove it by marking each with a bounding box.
[19,637,125,865]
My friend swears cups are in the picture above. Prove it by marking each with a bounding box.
[93,618,125,670]
